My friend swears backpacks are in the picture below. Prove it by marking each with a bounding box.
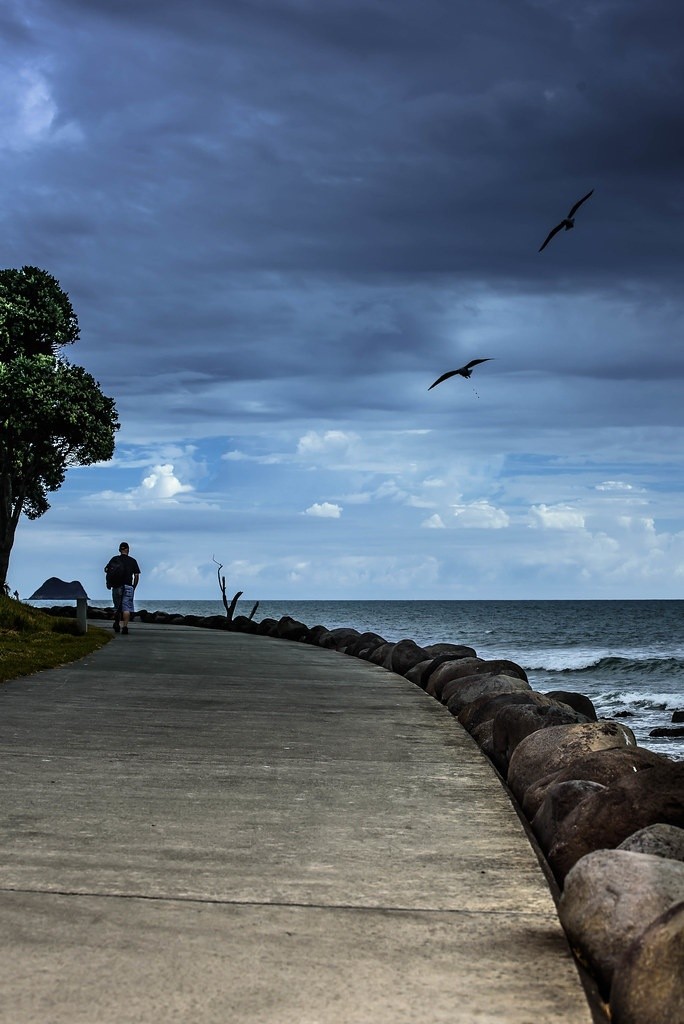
[107,556,123,587]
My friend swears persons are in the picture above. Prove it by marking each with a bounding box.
[103,542,142,636]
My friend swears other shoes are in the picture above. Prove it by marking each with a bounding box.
[113,622,121,634]
[121,627,128,635]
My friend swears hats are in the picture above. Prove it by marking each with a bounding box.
[119,542,129,550]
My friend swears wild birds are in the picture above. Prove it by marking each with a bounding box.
[532,187,601,254]
[425,350,497,393]
